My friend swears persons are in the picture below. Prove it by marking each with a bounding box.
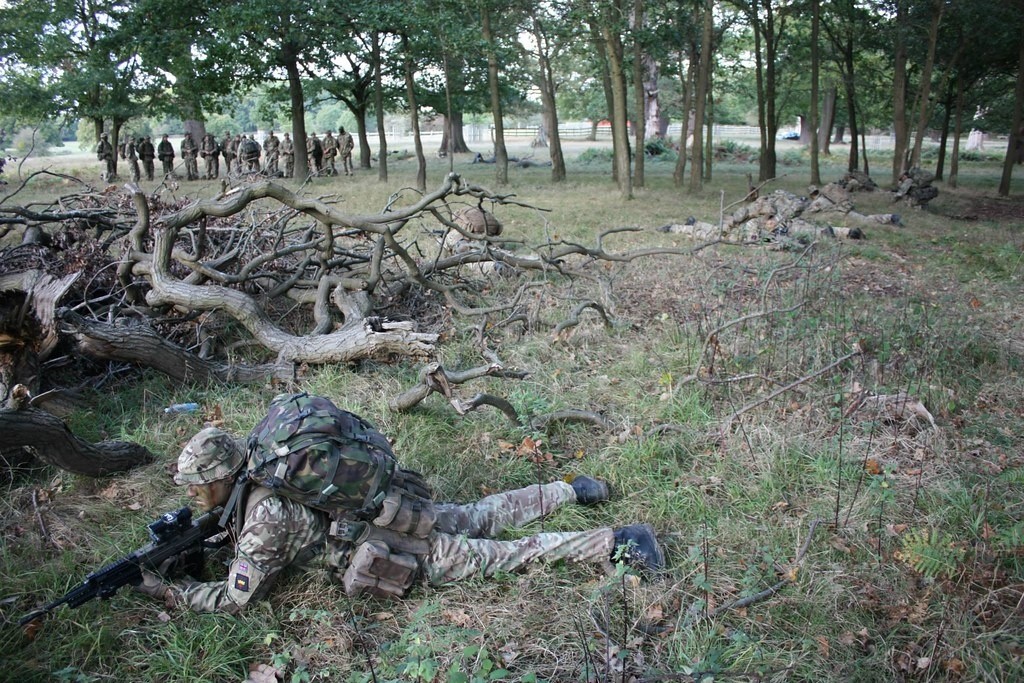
[659,208,867,251]
[890,169,929,210]
[181,130,198,180]
[278,133,294,177]
[436,223,566,279]
[97,132,115,175]
[305,130,337,171]
[124,136,140,182]
[158,134,174,178]
[263,130,281,174]
[336,126,355,176]
[135,427,666,616]
[221,131,262,172]
[200,134,218,180]
[843,172,890,193]
[807,185,900,226]
[139,135,154,181]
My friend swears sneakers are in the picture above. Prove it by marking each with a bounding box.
[571,474,609,507]
[611,521,667,584]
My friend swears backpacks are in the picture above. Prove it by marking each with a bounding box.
[813,183,854,212]
[907,168,937,190]
[96,143,103,161]
[763,189,806,219]
[242,139,259,161]
[848,168,871,184]
[451,206,504,238]
[215,391,398,584]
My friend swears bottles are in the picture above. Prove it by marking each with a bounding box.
[164,402,198,413]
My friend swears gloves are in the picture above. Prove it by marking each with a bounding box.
[890,187,899,204]
[125,561,168,600]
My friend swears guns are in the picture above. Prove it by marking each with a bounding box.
[421,229,445,237]
[760,229,806,251]
[18,506,230,625]
[832,179,849,189]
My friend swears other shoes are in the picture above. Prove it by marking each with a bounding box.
[655,215,695,231]
[493,260,527,279]
[345,172,353,176]
[817,226,867,241]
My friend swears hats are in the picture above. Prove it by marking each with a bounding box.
[172,427,247,487]
[310,125,344,136]
[184,130,254,136]
[128,133,169,140]
[808,183,818,195]
[100,132,108,138]
[843,171,853,178]
[897,171,907,181]
[268,130,290,136]
[773,213,786,225]
